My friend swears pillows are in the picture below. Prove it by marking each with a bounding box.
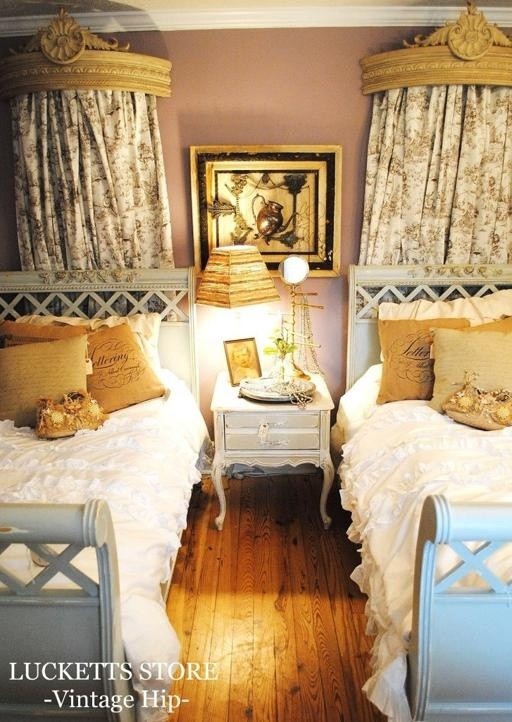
[375,317,471,405]
[429,328,512,426]
[470,316,512,333]
[1,321,88,350]
[87,323,166,414]
[0,333,89,428]
[379,289,512,365]
[15,313,162,384]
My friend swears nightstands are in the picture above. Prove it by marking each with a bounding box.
[210,371,335,530]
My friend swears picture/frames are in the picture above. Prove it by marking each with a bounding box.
[222,337,262,387]
[187,145,341,279]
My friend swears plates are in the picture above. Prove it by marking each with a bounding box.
[238,378,317,403]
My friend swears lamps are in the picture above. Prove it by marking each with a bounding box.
[196,243,278,308]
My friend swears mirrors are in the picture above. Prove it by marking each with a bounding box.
[278,255,311,287]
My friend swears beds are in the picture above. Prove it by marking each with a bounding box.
[332,265,512,722]
[1,269,205,721]
